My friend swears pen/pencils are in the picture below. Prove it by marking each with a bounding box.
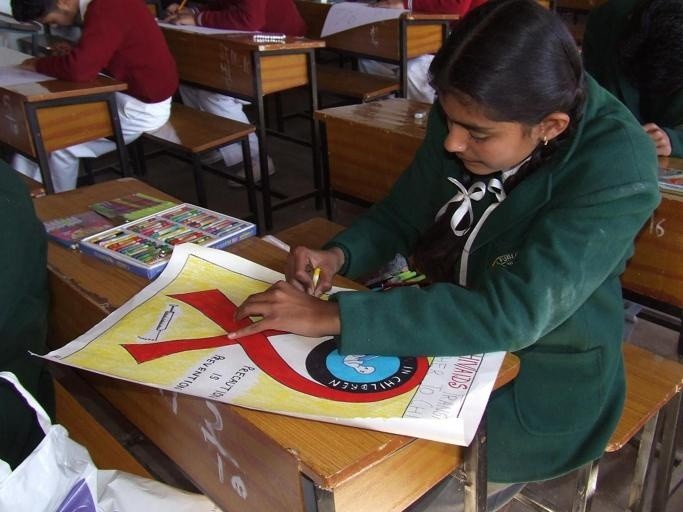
[41,193,178,249]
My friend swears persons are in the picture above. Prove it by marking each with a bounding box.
[358,0,488,105]
[581,0,683,157]
[162,0,308,187]
[10,0,181,193]
[227,0,662,512]
[0,161,56,470]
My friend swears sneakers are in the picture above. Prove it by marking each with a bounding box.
[199,150,276,188]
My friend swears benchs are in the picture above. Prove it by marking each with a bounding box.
[265,216,682,510]
[267,64,401,141]
[129,102,260,237]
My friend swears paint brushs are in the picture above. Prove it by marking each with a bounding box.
[312,265,321,297]
[175,0,186,12]
[78,203,257,279]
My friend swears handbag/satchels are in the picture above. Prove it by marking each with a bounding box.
[0,370,227,512]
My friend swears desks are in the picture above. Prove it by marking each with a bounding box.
[297,1,451,99]
[32,177,519,510]
[158,25,327,227]
[312,96,682,358]
[0,48,129,196]
